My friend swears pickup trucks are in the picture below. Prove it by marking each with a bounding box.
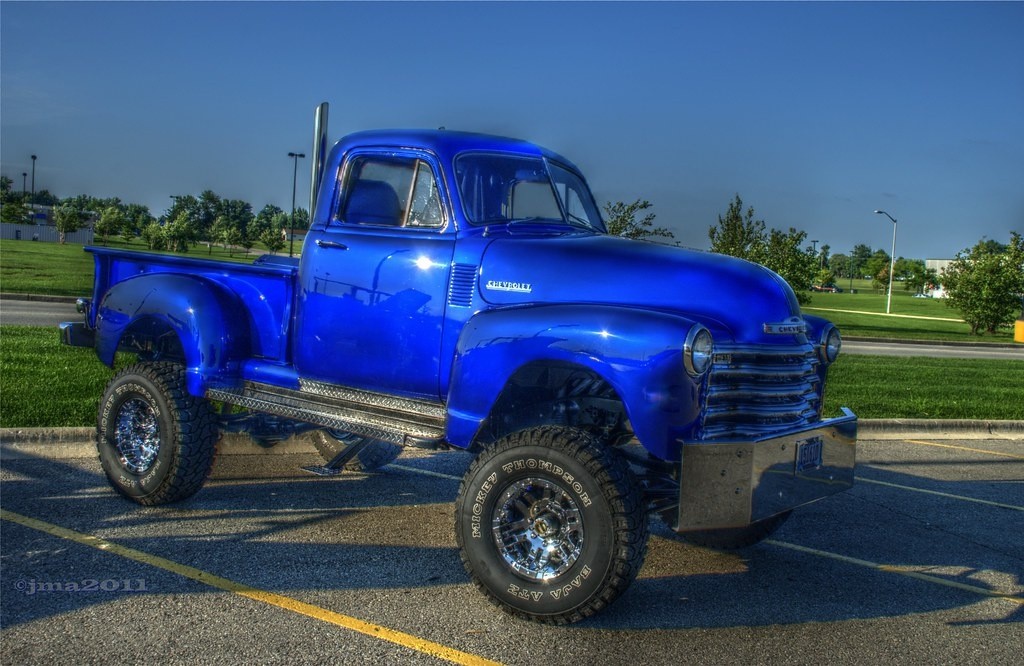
[58,102,858,626]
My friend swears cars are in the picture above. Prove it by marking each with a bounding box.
[809,283,843,293]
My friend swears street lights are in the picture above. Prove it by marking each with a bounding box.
[288,152,306,257]
[22,155,36,225]
[875,210,897,313]
[170,196,178,208]
[811,240,819,251]
[850,251,854,289]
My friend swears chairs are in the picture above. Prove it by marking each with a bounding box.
[343,180,402,227]
